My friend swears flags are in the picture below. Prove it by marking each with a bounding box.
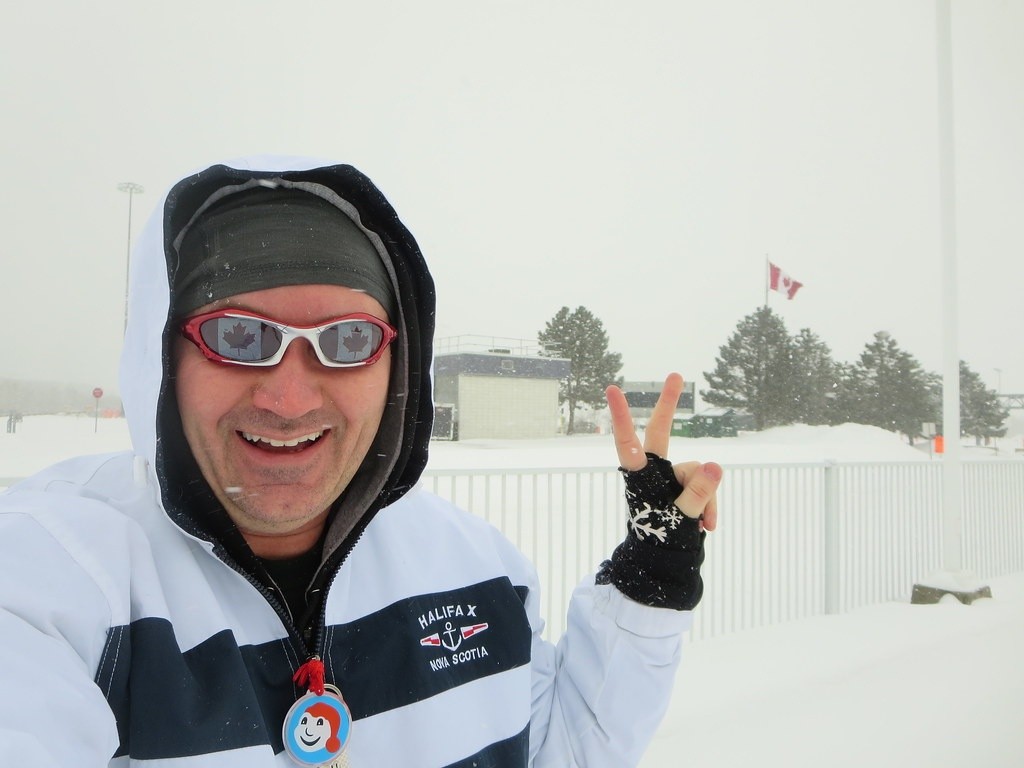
[768,261,804,302]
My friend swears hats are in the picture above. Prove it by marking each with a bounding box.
[175,185,398,329]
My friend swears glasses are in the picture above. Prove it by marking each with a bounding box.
[177,309,403,369]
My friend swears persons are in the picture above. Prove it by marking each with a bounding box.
[0,162,722,767]
